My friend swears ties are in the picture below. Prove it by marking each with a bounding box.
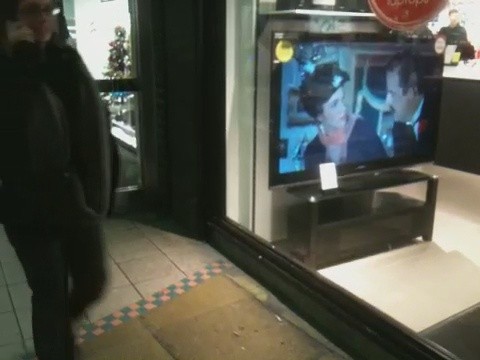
[404,118,420,163]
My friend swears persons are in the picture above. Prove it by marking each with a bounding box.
[438,9,467,45]
[299,61,388,171]
[385,49,433,155]
[0,1,113,360]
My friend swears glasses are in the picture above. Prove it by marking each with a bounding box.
[16,6,61,16]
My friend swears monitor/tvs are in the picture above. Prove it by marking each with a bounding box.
[269,29,446,191]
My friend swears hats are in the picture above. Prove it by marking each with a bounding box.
[300,62,350,101]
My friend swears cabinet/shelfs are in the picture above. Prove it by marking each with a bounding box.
[286,168,439,271]
